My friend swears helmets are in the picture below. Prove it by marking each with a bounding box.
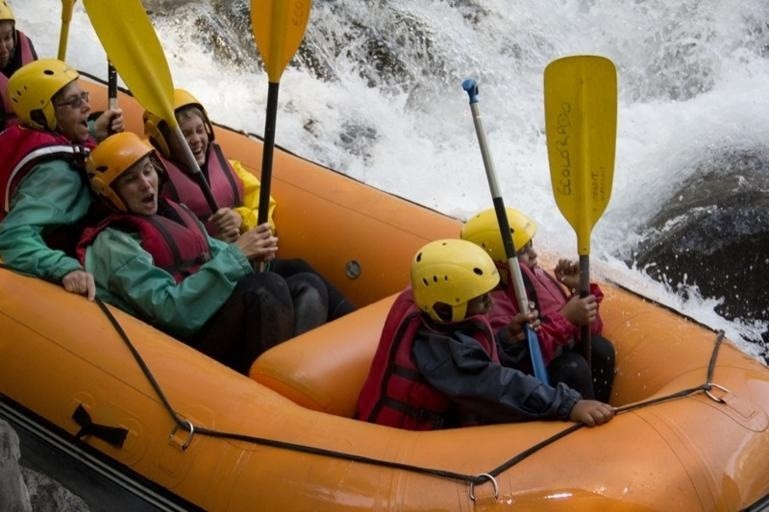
[87,133,169,212]
[0,0,16,21]
[413,239,501,323]
[460,207,536,263]
[9,58,80,130]
[143,89,215,157]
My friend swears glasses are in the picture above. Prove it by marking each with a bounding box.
[59,92,89,107]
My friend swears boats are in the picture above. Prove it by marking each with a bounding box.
[0,70,768,512]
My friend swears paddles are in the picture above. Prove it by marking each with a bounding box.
[463,80,550,387]
[82,1,219,212]
[250,0,315,273]
[543,56,617,371]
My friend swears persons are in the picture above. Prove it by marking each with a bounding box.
[76,131,330,377]
[141,88,354,321]
[459,208,615,404]
[1,58,126,301]
[0,1,39,128]
[356,238,615,430]
